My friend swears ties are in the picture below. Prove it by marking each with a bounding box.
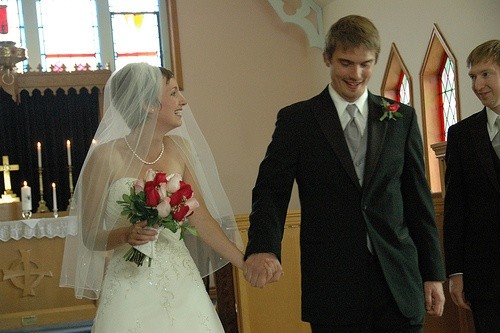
[345,104,373,255]
[493,117,500,159]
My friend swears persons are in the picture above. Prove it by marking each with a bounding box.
[442,39,500,333]
[80,62,284,333]
[243,14,446,333]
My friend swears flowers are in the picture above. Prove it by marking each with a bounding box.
[379,97,403,123]
[117,169,200,270]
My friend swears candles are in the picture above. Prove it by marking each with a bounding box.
[20,179,33,212]
[51,182,58,209]
[66,140,72,165]
[36,141,43,168]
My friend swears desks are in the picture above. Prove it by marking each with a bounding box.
[0,202,99,329]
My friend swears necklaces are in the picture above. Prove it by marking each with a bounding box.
[124,135,164,164]
